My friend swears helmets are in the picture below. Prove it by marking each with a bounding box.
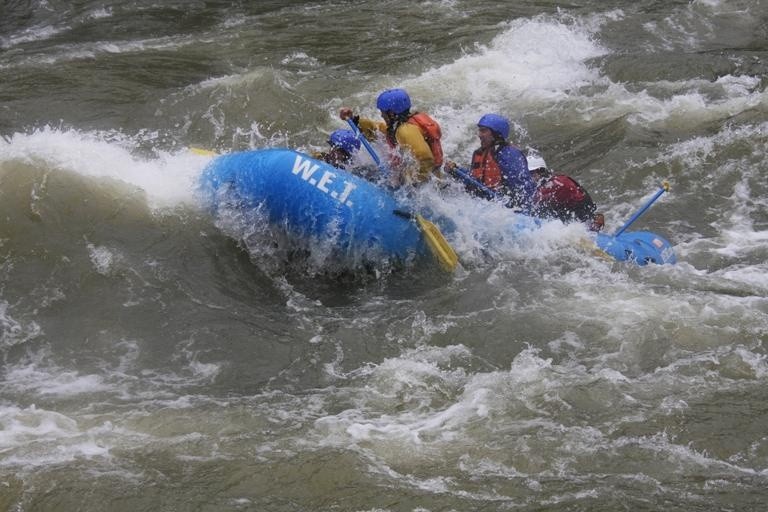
[526,154,546,171]
[476,113,510,138]
[329,129,361,157]
[377,88,411,114]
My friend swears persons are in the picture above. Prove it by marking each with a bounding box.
[526,154,604,232]
[312,128,359,180]
[341,88,446,201]
[445,114,532,217]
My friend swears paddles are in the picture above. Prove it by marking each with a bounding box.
[346,115,457,268]
[451,169,618,263]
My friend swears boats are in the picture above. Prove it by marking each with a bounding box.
[200,147,677,267]
